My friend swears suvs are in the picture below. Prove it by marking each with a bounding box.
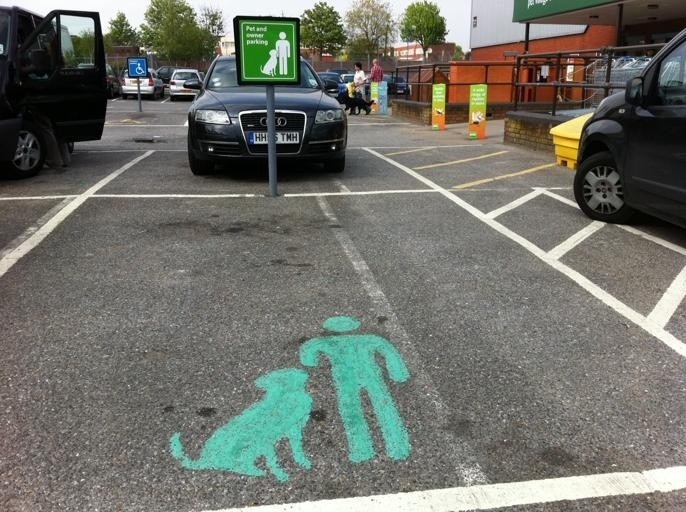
[0,6,108,182]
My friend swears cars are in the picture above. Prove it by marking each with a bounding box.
[315,67,409,105]
[183,54,349,176]
[74,61,206,103]
[574,28,686,232]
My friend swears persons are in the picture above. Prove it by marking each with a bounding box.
[352,60,371,115]
[275,31,291,76]
[38,116,71,169]
[135,62,144,75]
[298,315,414,464]
[367,57,382,82]
